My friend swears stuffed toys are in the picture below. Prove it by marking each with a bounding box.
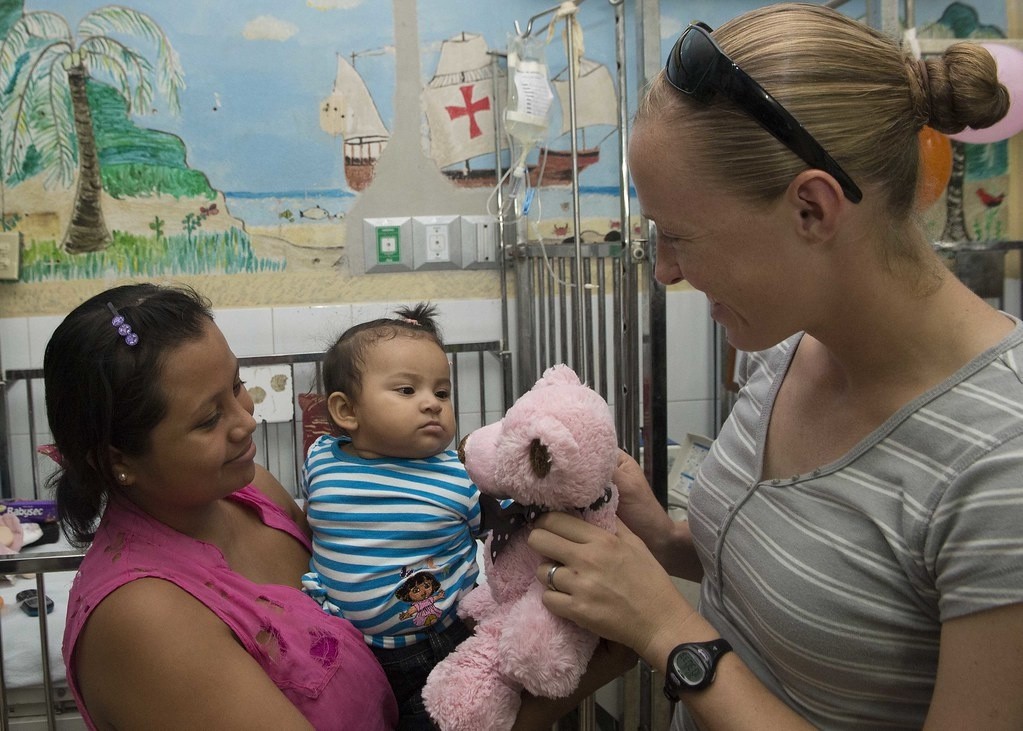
[420,363,619,731]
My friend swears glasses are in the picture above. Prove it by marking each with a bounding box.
[667,22,868,205]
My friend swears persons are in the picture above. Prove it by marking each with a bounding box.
[300,297,513,731]
[527,3,1023,731]
[43,283,399,731]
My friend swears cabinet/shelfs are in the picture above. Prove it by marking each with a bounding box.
[0,341,501,731]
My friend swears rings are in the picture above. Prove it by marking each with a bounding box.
[546,564,563,592]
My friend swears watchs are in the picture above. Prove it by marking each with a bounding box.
[662,638,733,704]
